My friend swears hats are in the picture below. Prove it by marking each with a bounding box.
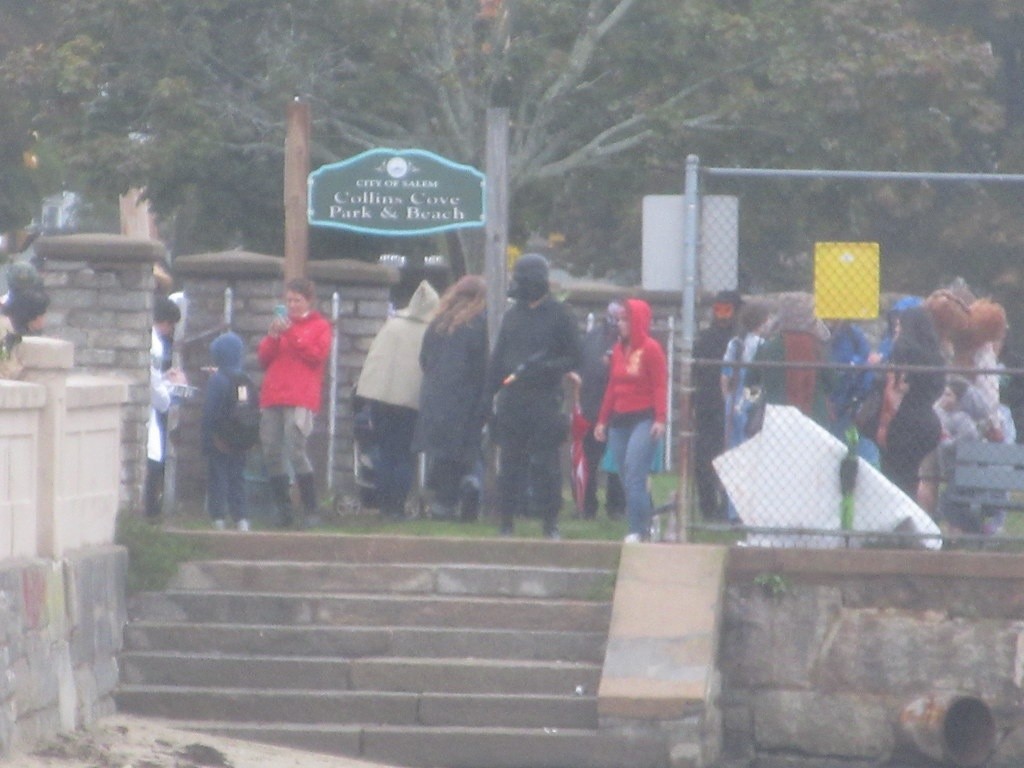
[710,290,742,306]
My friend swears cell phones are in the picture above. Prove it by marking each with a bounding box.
[273,304,286,319]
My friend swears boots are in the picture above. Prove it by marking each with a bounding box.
[271,473,293,528]
[297,473,317,530]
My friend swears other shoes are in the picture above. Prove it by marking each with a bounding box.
[213,520,224,530]
[500,524,515,535]
[543,527,558,538]
[623,534,643,543]
[237,520,250,531]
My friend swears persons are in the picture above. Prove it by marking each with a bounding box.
[256,278,331,528]
[351,253,667,541]
[0,261,49,366]
[199,332,251,533]
[690,288,1016,547]
[144,266,185,517]
